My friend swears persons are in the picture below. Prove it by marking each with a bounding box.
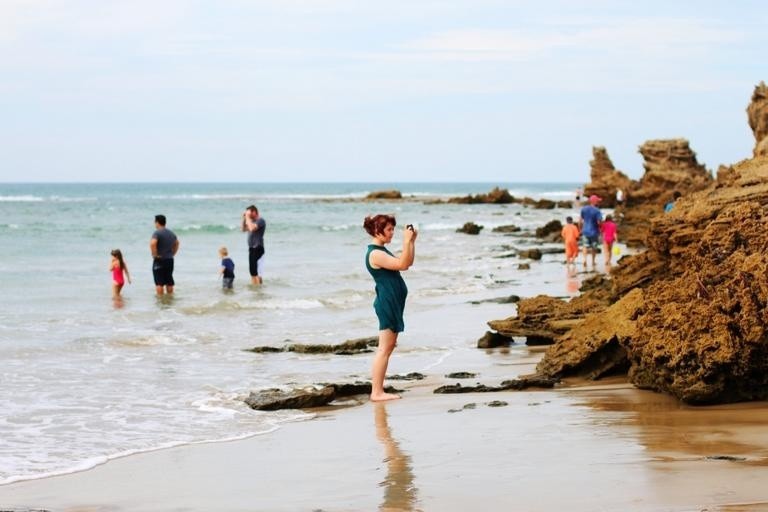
[561,195,617,267]
[150,215,178,295]
[576,187,584,209]
[664,191,681,213]
[110,249,131,300]
[363,214,417,402]
[240,205,265,284]
[218,246,234,288]
[615,187,624,210]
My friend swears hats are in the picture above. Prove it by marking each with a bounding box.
[590,195,603,202]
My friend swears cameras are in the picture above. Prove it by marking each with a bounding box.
[407,224,414,233]
